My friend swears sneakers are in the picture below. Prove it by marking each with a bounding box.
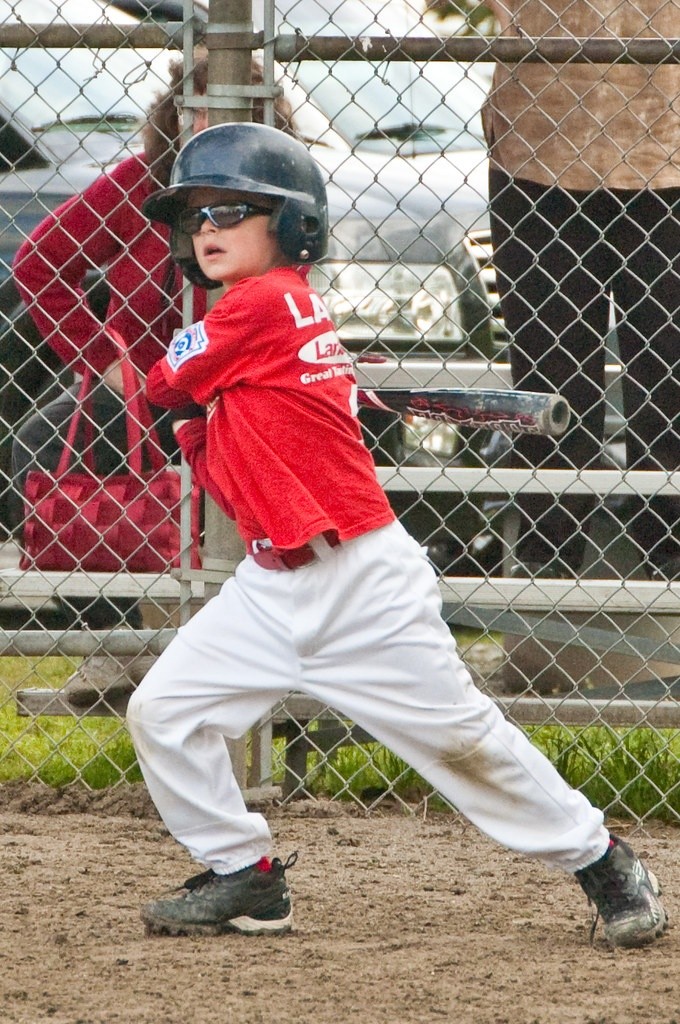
[140,852,298,939]
[65,621,161,707]
[574,834,669,949]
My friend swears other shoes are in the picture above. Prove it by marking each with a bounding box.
[651,553,680,581]
[510,561,572,579]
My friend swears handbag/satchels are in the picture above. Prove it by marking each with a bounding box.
[20,326,201,573]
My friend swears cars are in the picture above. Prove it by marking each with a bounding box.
[2,0,628,575]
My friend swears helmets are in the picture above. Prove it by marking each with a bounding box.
[141,122,327,290]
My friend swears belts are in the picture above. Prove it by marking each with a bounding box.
[249,528,340,569]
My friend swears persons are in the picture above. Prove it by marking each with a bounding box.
[12,59,300,703]
[125,121,668,953]
[479,0,680,583]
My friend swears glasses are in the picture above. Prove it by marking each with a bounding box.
[176,104,210,131]
[177,201,273,235]
